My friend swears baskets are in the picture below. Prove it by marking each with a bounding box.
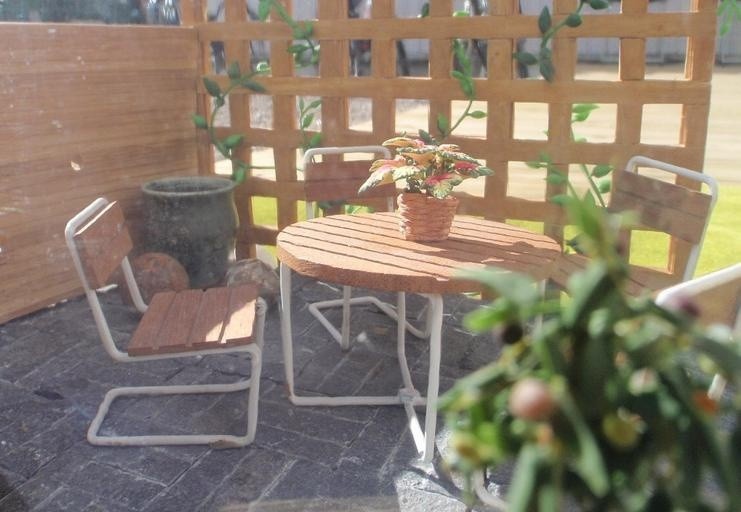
[397,190,458,241]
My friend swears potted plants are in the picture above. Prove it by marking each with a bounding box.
[356,128,498,242]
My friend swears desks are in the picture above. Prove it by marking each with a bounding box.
[277,208,565,460]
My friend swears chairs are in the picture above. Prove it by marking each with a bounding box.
[62,197,270,446]
[302,143,436,350]
[548,156,740,399]
